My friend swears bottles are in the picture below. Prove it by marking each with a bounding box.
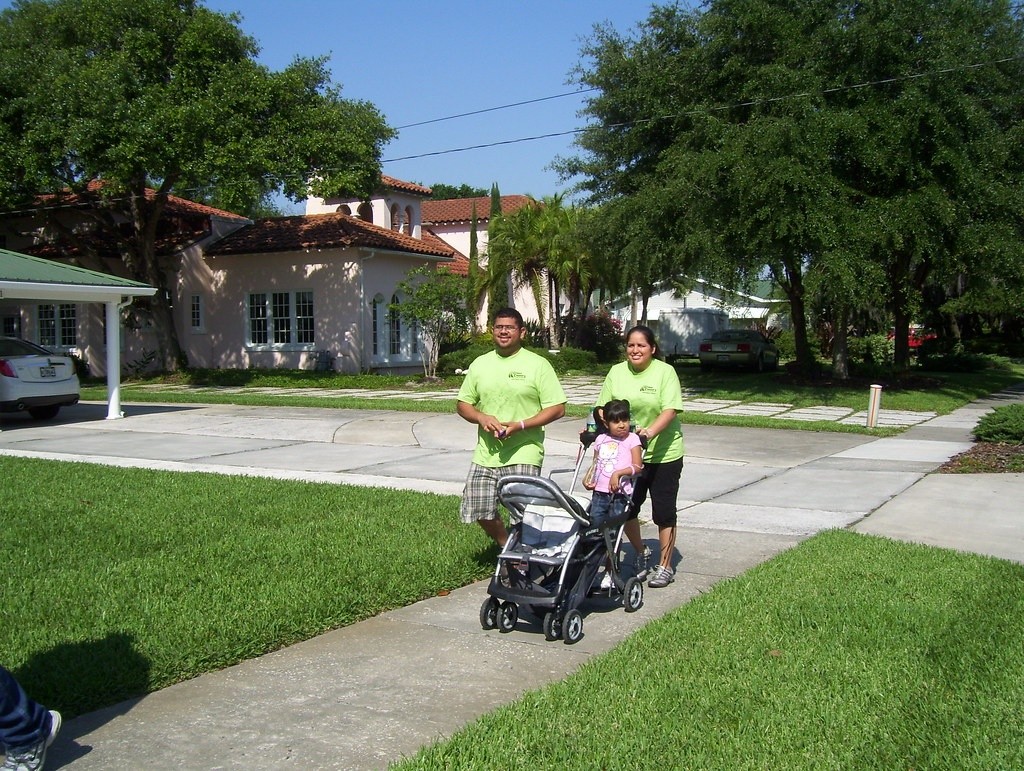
[587,407,597,433]
[629,413,636,433]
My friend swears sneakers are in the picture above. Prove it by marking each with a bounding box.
[0,710,61,771]
[633,543,653,571]
[648,565,674,587]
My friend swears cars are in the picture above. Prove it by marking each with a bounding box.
[887,317,937,349]
[698,329,780,374]
[0,335,81,423]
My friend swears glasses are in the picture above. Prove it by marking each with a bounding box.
[492,325,519,332]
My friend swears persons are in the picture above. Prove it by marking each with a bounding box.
[589,399,643,589]
[456,309,567,582]
[580,325,684,588]
[0,664,61,771]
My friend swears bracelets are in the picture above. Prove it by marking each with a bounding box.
[520,420,524,430]
[631,466,635,476]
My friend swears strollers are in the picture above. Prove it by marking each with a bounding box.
[479,430,650,644]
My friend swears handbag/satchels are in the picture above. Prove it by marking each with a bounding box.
[605,488,635,527]
